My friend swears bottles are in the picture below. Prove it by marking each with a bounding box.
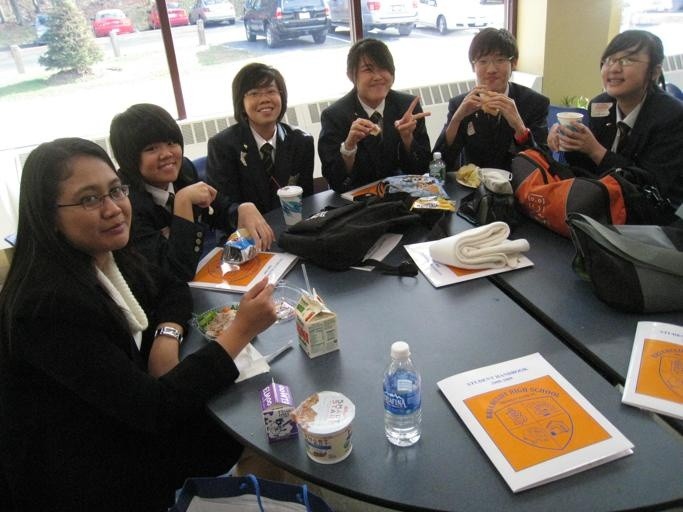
[383,341,422,448]
[428,152,447,186]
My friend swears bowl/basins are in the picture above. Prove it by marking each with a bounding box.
[188,279,309,343]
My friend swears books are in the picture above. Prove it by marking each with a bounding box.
[436,351,635,495]
[621,320,683,421]
[402,234,536,289]
[187,247,299,293]
[340,179,385,202]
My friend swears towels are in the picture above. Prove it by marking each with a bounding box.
[429,221,529,270]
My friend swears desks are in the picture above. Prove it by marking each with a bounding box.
[180,172,683,512]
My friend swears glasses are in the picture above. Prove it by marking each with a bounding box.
[472,55,514,67]
[244,88,283,97]
[57,185,130,210]
[602,56,650,65]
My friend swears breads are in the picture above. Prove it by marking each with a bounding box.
[479,89,503,116]
[456,163,482,187]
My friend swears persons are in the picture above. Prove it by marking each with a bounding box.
[110,103,276,291]
[205,63,314,243]
[1,138,278,511]
[546,30,683,223]
[318,39,432,194]
[431,27,550,170]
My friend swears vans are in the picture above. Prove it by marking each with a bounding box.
[330,1,418,37]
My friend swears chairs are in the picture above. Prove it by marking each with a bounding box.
[547,106,590,163]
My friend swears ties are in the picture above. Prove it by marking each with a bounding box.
[369,112,382,145]
[166,193,175,214]
[260,143,275,178]
[616,122,630,153]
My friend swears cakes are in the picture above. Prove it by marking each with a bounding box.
[199,307,240,340]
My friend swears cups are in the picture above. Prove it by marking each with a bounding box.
[556,112,584,151]
[277,185,303,226]
[296,391,357,466]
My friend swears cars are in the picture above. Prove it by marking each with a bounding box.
[620,2,661,27]
[147,2,189,29]
[91,7,134,38]
[243,1,329,45]
[418,1,490,33]
[190,1,239,24]
[33,13,56,44]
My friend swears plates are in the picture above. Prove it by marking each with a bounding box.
[456,168,513,189]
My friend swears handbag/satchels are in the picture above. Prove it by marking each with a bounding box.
[565,212,683,314]
[169,474,333,512]
[511,149,678,237]
[277,192,421,272]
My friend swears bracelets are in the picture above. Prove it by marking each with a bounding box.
[514,128,531,145]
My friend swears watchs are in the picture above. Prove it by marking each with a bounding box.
[339,142,358,157]
[154,326,184,344]
